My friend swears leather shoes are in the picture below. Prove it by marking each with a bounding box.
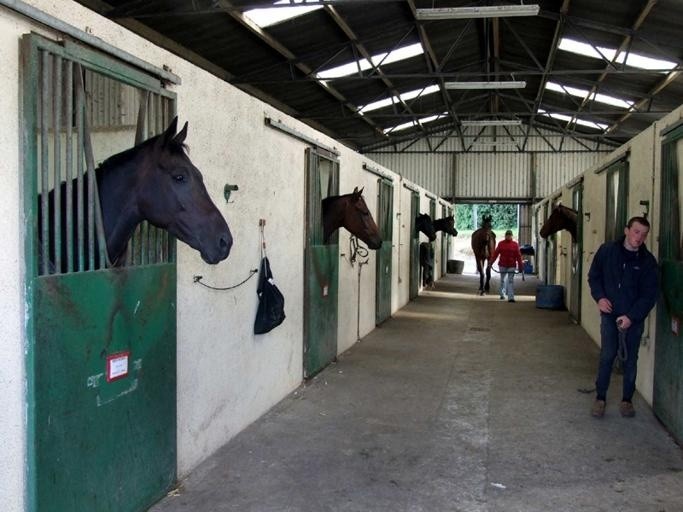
[619,401,635,417]
[592,400,606,417]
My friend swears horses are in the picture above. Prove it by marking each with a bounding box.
[539,202,578,243]
[321,185,383,251]
[415,213,458,242]
[471,215,496,296]
[37,114,233,277]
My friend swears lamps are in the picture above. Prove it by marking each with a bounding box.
[462,116,522,146]
[415,0,540,20]
[444,74,527,89]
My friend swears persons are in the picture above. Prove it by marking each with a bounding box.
[588,217,663,421]
[487,229,525,302]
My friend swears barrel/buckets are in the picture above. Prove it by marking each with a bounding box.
[536,284,564,311]
[447,260,464,274]
